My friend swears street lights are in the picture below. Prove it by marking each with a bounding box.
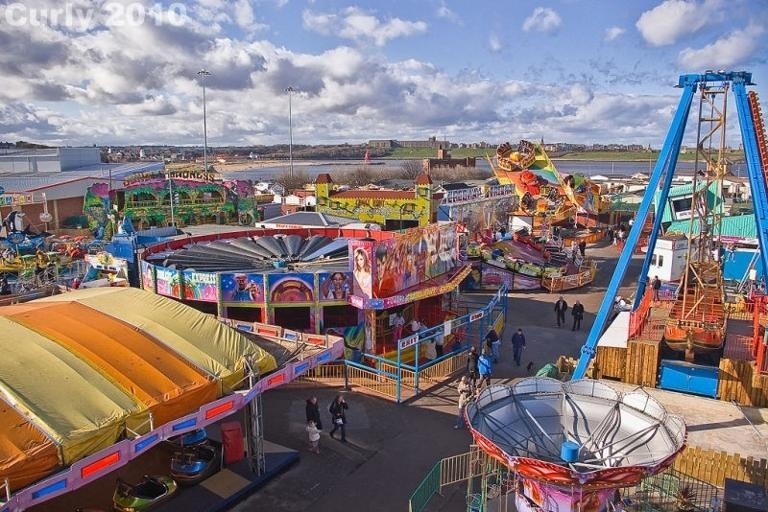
[196,68,213,181]
[707,83,723,182]
[283,86,297,178]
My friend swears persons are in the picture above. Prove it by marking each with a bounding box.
[392,310,443,362]
[352,247,372,295]
[228,274,261,301]
[652,275,661,301]
[605,216,633,248]
[328,395,350,445]
[497,224,587,264]
[306,420,322,455]
[372,245,395,297]
[512,328,526,368]
[642,276,650,298]
[453,325,501,430]
[554,295,568,327]
[571,299,585,331]
[304,394,323,445]
[322,271,353,300]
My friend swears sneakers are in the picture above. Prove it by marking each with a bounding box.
[328,432,348,443]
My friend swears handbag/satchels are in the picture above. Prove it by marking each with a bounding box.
[331,414,346,426]
